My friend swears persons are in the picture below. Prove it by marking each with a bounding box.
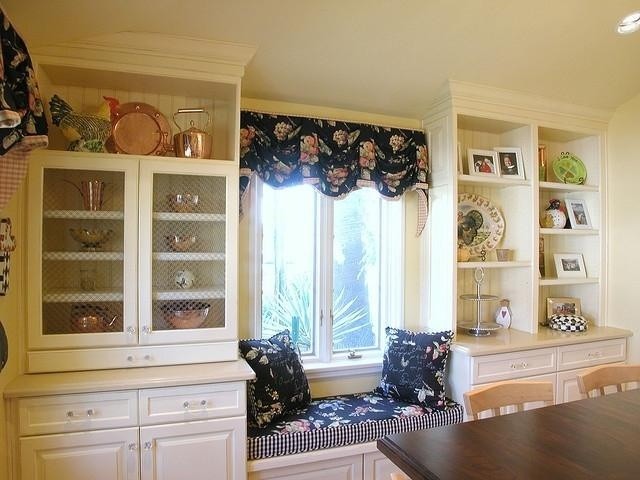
[503,155,516,173]
[553,304,575,315]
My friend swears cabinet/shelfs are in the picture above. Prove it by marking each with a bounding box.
[441,327,633,421]
[248,443,399,480]
[20,41,257,375]
[535,110,610,335]
[418,97,537,340]
[3,357,255,480]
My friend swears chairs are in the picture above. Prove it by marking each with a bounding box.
[576,362,640,399]
[464,382,554,422]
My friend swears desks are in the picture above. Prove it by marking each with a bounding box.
[378,385,639,480]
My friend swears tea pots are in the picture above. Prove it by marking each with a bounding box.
[70,304,119,333]
[162,106,214,159]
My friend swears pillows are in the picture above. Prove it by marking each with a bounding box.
[373,326,454,410]
[237,327,312,430]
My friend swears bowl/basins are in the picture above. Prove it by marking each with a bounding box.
[167,235,196,252]
[162,302,212,329]
[69,227,112,252]
[168,194,199,212]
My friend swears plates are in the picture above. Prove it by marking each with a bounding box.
[457,193,505,258]
[553,154,587,185]
[105,102,172,156]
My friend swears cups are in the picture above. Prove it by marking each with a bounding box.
[80,270,97,291]
[496,248,510,262]
[82,181,105,211]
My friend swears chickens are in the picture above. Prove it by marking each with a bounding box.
[49,95,120,153]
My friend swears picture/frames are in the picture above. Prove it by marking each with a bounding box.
[565,199,593,230]
[546,297,581,322]
[494,147,525,179]
[466,148,500,176]
[553,254,586,279]
